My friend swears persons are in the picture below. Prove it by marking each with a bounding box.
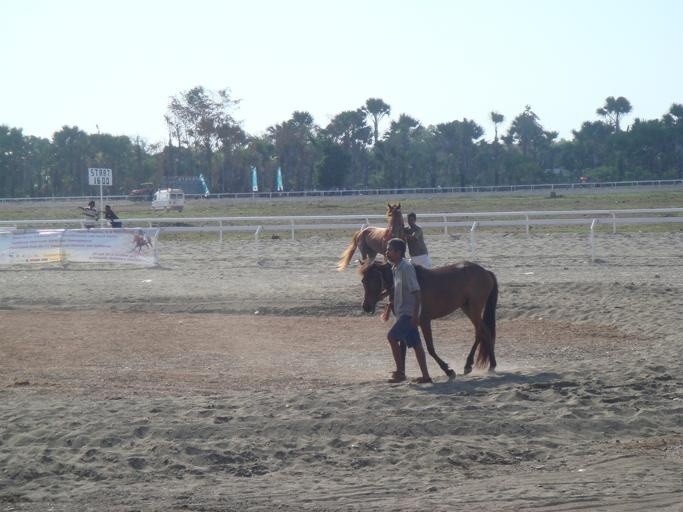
[401,212,431,268]
[76,200,98,229]
[99,204,122,229]
[378,237,434,388]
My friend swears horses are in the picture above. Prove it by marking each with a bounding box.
[337,202,405,272]
[129,234,152,253]
[357,258,497,382]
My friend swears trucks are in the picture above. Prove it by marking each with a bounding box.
[129,176,206,200]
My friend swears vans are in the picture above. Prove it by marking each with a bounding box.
[151,187,185,212]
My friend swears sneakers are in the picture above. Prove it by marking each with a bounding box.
[388,371,406,383]
[417,377,433,382]
